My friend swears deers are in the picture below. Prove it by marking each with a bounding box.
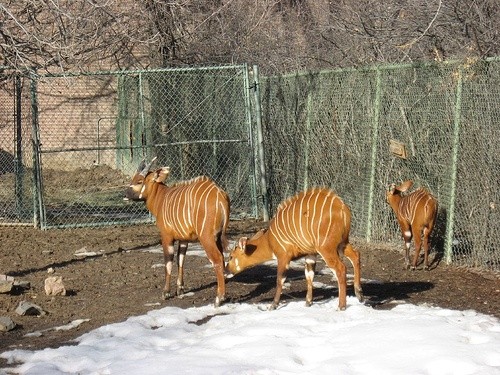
[126,154,231,307]
[382,181,440,272]
[223,187,364,311]
